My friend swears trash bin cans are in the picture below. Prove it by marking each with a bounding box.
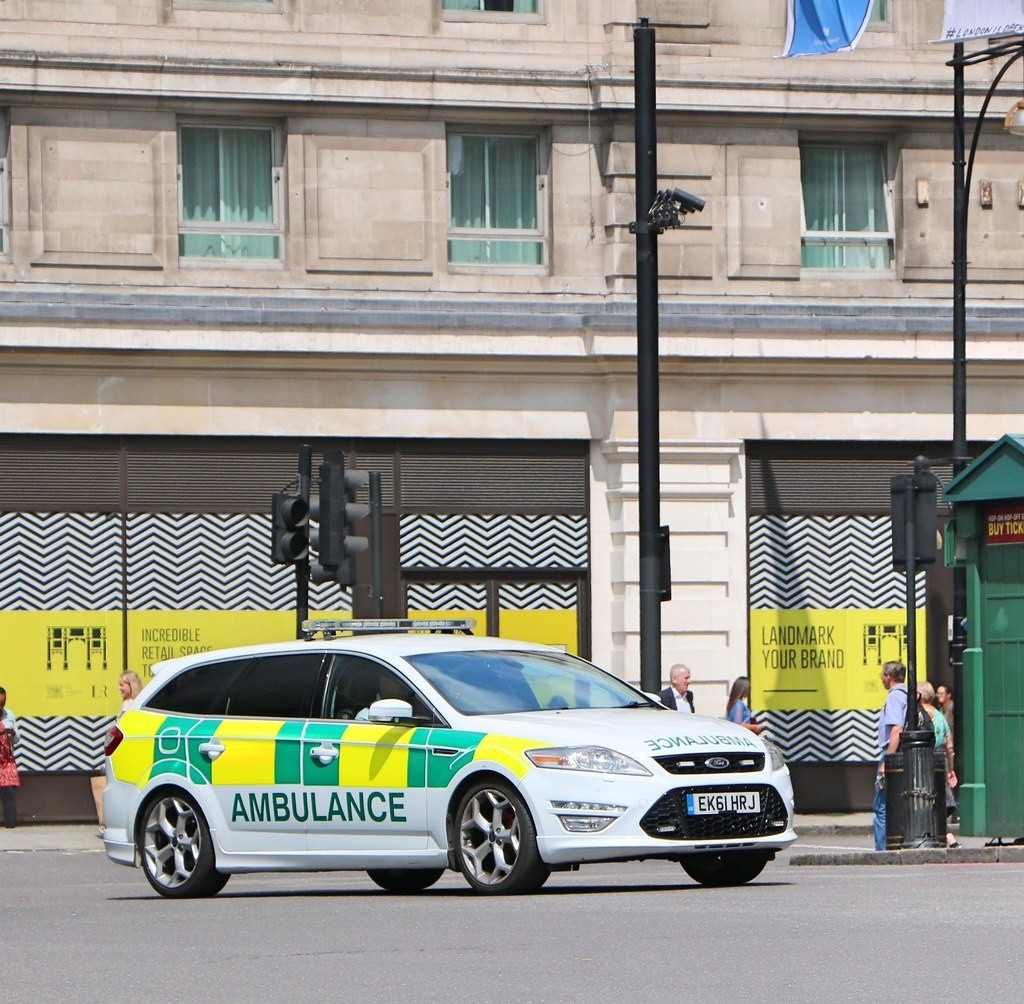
[885,750,949,850]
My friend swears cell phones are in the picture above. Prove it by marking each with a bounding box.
[4,728,9,732]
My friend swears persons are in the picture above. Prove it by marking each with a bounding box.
[725,677,766,736]
[0,687,20,828]
[873,661,959,851]
[356,671,414,722]
[655,664,695,714]
[98,671,144,836]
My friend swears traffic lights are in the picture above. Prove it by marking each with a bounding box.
[272,453,370,592]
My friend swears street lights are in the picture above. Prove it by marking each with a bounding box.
[945,40,1023,824]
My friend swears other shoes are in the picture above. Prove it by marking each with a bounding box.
[946,833,958,847]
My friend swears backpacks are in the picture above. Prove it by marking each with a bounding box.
[884,689,934,732]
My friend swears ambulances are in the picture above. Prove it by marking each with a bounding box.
[103,617,796,900]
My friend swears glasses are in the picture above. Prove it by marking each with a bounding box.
[936,692,943,696]
